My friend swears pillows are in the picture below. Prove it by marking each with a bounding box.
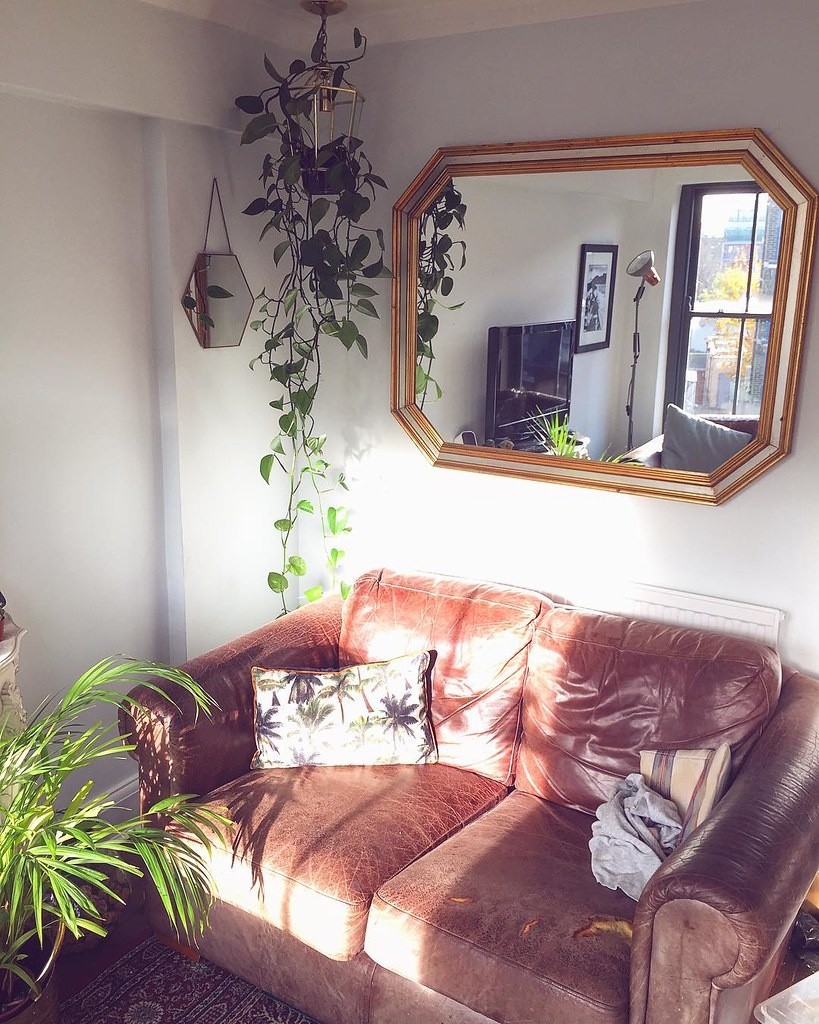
[252,651,438,769]
[661,403,753,474]
[639,743,731,848]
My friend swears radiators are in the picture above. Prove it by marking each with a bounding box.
[615,583,787,652]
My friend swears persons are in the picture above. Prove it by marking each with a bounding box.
[585,283,602,332]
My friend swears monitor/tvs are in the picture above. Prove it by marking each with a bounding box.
[487,319,583,452]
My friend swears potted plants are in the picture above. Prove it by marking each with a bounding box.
[234,40,389,620]
[0,655,237,1024]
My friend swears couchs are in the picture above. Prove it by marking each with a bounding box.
[119,570,819,1024]
[615,415,760,469]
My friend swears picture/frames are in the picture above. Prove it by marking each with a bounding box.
[575,244,619,351]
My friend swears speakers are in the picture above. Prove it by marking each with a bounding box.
[454,431,477,446]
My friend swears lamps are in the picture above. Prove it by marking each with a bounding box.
[624,249,662,450]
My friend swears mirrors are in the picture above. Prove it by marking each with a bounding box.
[181,178,255,349]
[392,126,819,508]
